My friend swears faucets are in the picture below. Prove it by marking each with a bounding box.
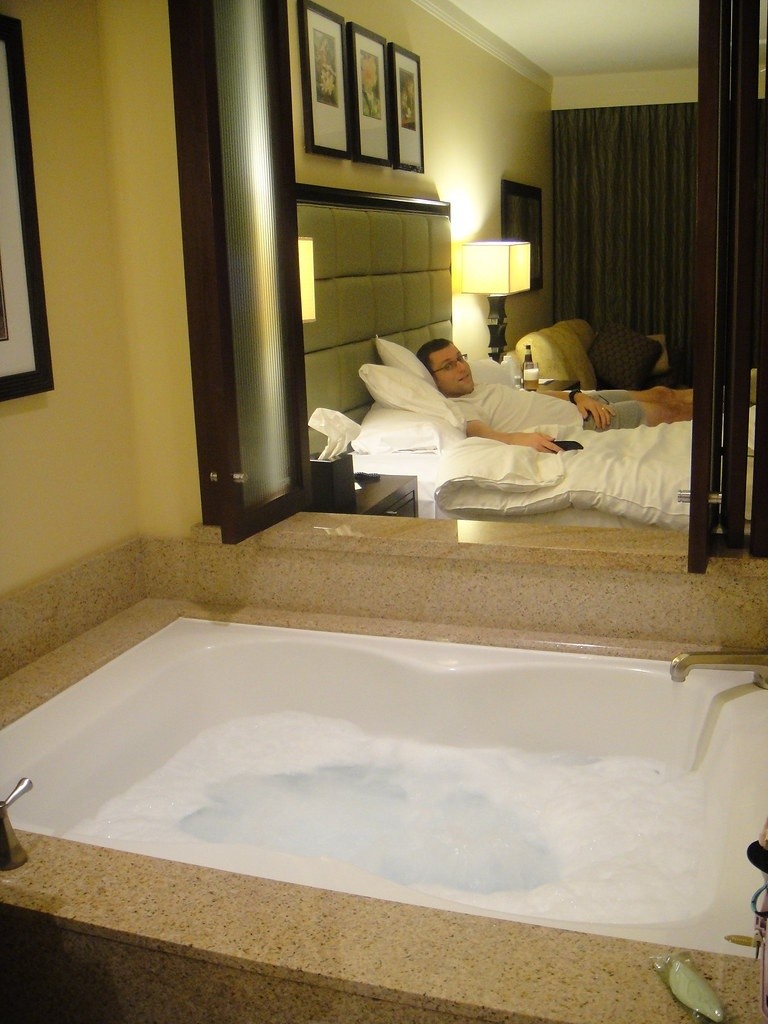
[670,649,768,690]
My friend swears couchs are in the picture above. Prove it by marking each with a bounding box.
[515,318,670,392]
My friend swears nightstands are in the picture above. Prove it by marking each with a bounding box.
[353,473,418,517]
[538,379,580,393]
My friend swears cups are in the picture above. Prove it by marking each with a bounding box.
[512,363,521,387]
[524,362,539,391]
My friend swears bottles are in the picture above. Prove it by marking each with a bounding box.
[501,356,514,385]
[522,346,534,388]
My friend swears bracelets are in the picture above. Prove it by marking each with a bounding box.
[569,389,582,405]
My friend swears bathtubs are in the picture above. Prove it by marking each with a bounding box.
[0,613,768,968]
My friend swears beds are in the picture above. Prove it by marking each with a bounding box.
[296,183,756,532]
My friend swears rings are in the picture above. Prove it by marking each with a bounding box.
[602,406,605,410]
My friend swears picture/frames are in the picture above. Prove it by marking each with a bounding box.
[500,178,543,289]
[297,0,352,159]
[386,42,424,173]
[0,15,55,403]
[345,20,393,165]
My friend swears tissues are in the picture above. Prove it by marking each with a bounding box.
[304,401,361,512]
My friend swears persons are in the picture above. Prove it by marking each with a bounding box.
[416,339,755,454]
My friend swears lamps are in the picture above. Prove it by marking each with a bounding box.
[461,241,531,361]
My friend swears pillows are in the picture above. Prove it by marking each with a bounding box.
[588,323,664,391]
[376,335,439,388]
[358,363,463,427]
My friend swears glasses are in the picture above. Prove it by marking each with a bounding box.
[431,353,468,375]
